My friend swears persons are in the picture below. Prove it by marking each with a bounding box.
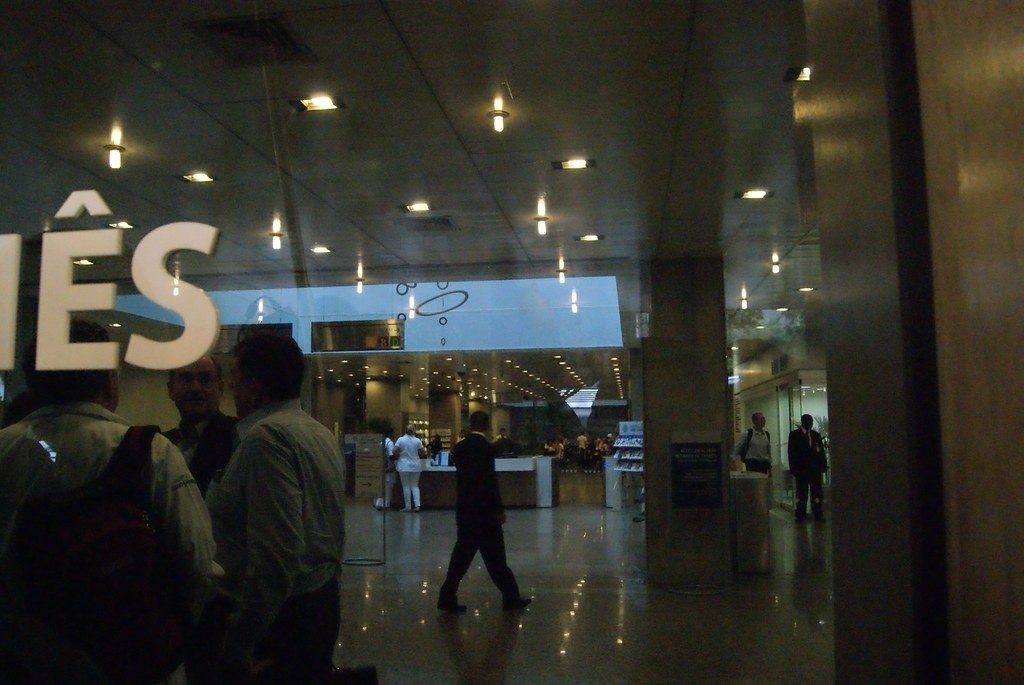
[544,429,614,468]
[788,415,828,522]
[461,425,461,427]
[0,321,218,685]
[736,412,772,473]
[455,429,465,444]
[187,330,347,685]
[437,410,533,612]
[394,425,426,512]
[158,350,240,491]
[490,426,507,441]
[381,428,399,508]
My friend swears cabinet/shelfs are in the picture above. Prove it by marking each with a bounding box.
[614,433,644,472]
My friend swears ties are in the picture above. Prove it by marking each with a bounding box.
[805,429,810,444]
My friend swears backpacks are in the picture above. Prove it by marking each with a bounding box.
[10,423,197,685]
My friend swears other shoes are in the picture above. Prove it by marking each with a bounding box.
[436,602,468,615]
[414,506,420,511]
[815,515,827,523]
[502,595,532,610]
[400,507,411,512]
